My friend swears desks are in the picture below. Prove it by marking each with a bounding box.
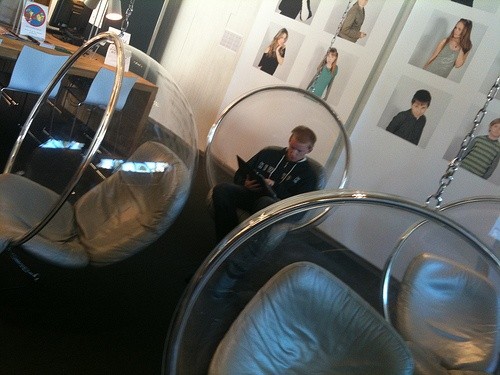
[0,20,159,157]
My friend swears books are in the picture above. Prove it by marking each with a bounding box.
[27,36,55,49]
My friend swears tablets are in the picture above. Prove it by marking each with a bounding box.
[237,155,277,199]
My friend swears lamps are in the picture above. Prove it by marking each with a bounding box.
[83,0,122,57]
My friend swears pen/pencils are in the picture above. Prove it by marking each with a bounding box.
[26,35,40,45]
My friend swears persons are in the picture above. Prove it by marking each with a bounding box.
[259,0,500,180]
[211,125,317,245]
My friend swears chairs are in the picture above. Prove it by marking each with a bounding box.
[205,87,351,256]
[0,32,199,297]
[49,67,137,158]
[161,189,499,375]
[379,197,500,375]
[0,45,70,136]
